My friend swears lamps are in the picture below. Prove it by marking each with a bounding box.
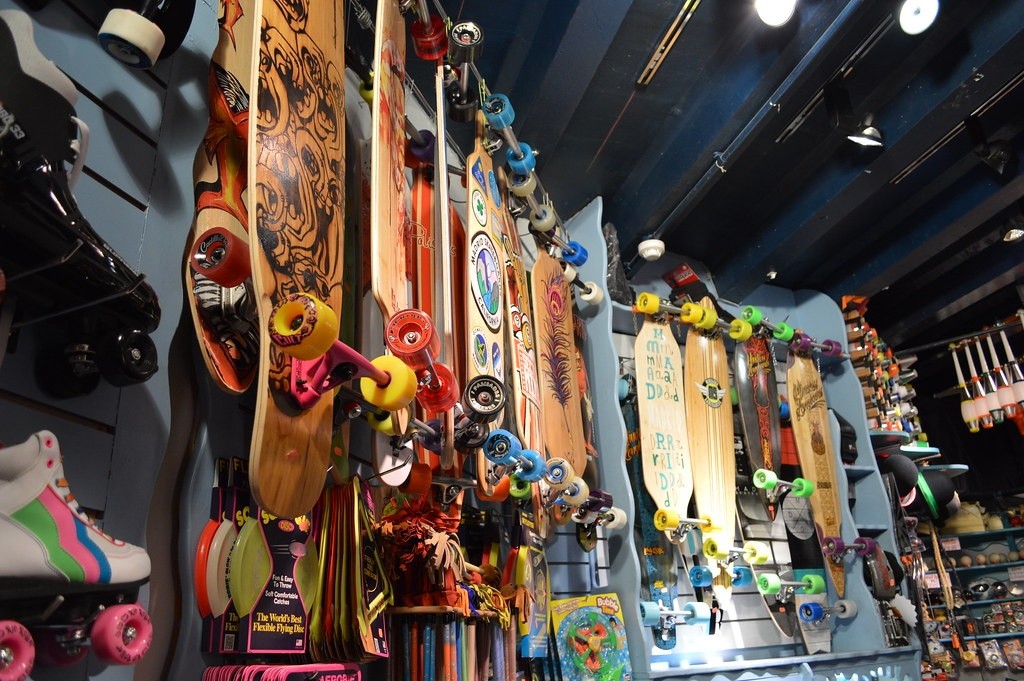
[845,124,886,148]
[974,140,1012,174]
[890,0,940,37]
[1000,215,1024,247]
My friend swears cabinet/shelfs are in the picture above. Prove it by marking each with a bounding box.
[923,527,1024,642]
[843,464,888,539]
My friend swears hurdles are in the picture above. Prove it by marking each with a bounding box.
[465,96,602,524]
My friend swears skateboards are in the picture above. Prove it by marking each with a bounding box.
[633,292,877,640]
[186,0,257,393]
[371,0,460,485]
[247,0,417,519]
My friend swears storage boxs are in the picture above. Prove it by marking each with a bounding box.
[977,639,1006,670]
[959,640,981,669]
[1003,640,1024,670]
